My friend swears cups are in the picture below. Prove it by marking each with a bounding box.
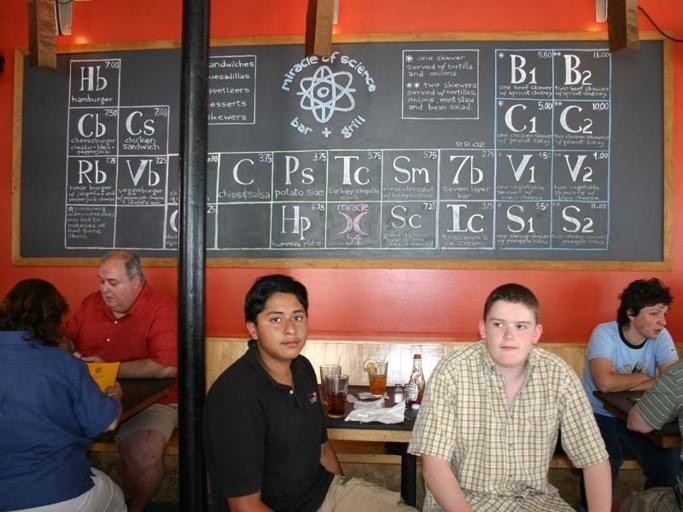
[368,360,390,395]
[325,374,349,419]
[319,364,342,405]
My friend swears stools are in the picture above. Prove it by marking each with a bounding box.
[87,419,178,459]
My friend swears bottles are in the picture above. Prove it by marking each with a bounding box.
[407,353,425,409]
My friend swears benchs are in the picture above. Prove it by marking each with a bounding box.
[205,336,683,470]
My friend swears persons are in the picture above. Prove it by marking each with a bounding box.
[619,360,683,512]
[407,282,612,512]
[0,277,128,512]
[205,274,420,512]
[579,278,679,508]
[59,250,177,512]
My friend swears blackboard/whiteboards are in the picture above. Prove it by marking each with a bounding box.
[12,30,672,273]
